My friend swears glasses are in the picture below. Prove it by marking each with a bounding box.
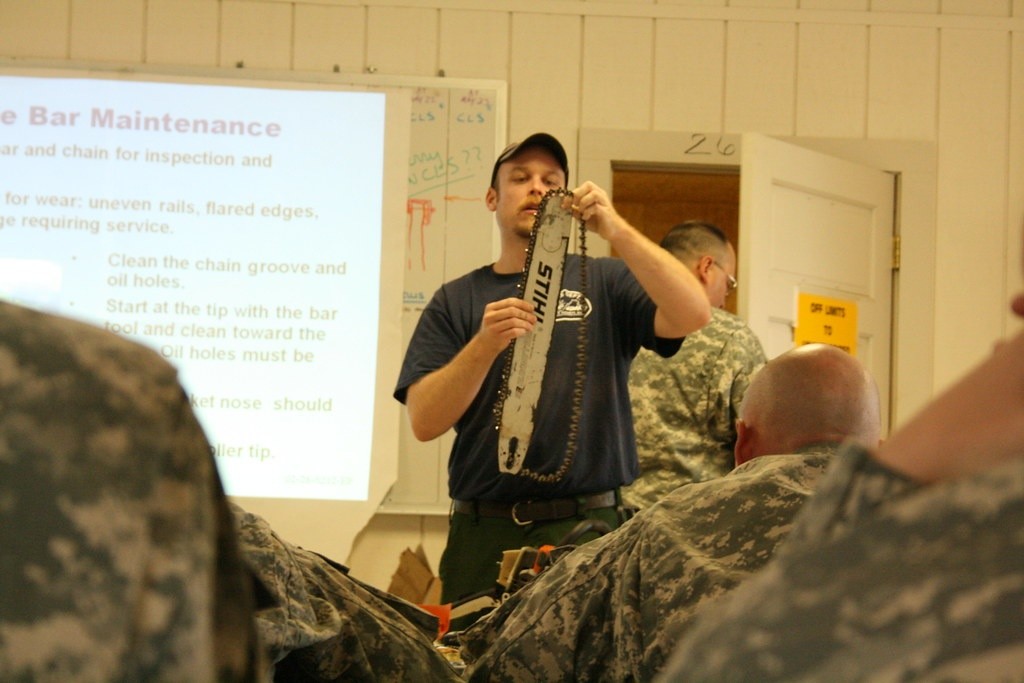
[698,256,737,298]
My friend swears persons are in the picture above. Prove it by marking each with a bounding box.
[393,131,711,605]
[0,219,1024,683]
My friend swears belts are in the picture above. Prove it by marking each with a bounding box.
[454,490,616,525]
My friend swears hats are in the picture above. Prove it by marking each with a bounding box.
[491,131,569,190]
